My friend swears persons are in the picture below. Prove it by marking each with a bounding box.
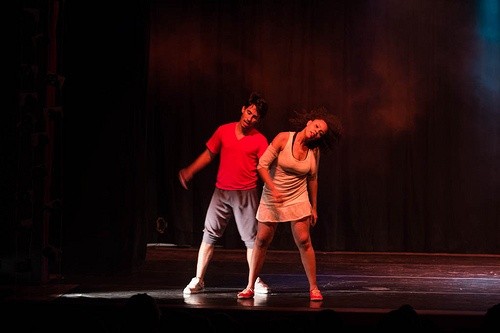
[237,106,344,301]
[176,95,269,295]
[0,256,500,333]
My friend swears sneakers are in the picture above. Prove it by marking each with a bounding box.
[254,276,270,293]
[183,294,208,305]
[238,299,254,307]
[310,301,322,308]
[238,287,254,298]
[183,277,204,294]
[253,293,268,306]
[310,288,323,300]
